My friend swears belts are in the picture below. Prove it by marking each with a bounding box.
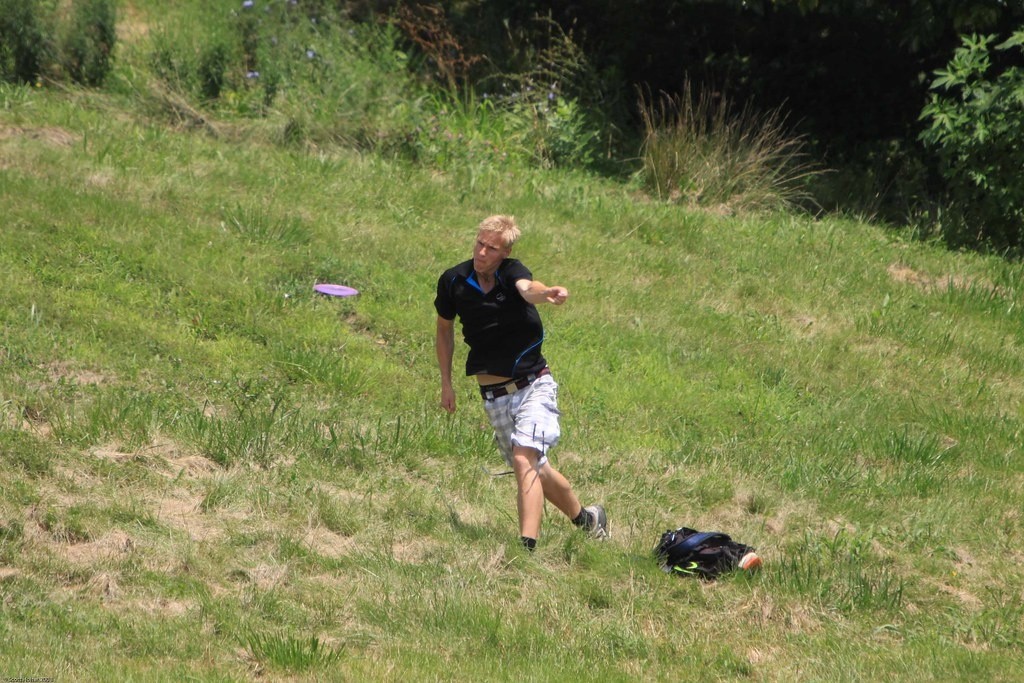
[481,368,551,400]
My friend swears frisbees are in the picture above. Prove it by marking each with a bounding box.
[314,282,358,295]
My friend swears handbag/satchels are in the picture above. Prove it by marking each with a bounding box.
[652,527,762,583]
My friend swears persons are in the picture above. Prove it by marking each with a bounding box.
[434,214,607,554]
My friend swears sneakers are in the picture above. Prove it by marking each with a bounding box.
[580,504,607,543]
